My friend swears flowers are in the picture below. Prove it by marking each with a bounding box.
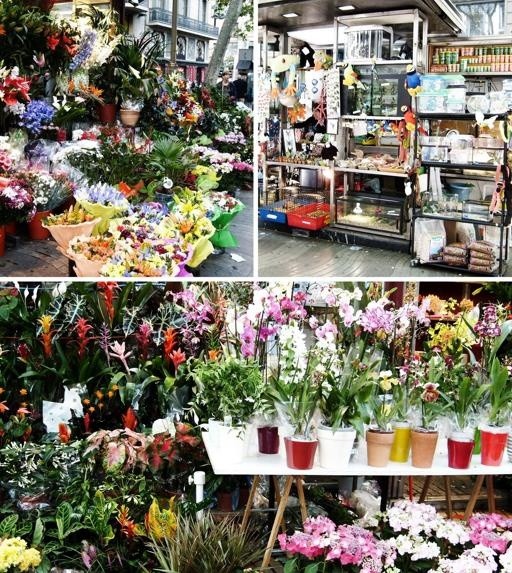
[0,2,253,276]
[0,282,512,573]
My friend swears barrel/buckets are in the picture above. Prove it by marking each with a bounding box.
[450,182,475,202]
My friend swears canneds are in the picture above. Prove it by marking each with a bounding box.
[431,46,512,72]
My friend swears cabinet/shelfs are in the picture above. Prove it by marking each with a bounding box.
[257,2,512,276]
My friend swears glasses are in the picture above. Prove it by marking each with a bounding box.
[312,79,318,94]
